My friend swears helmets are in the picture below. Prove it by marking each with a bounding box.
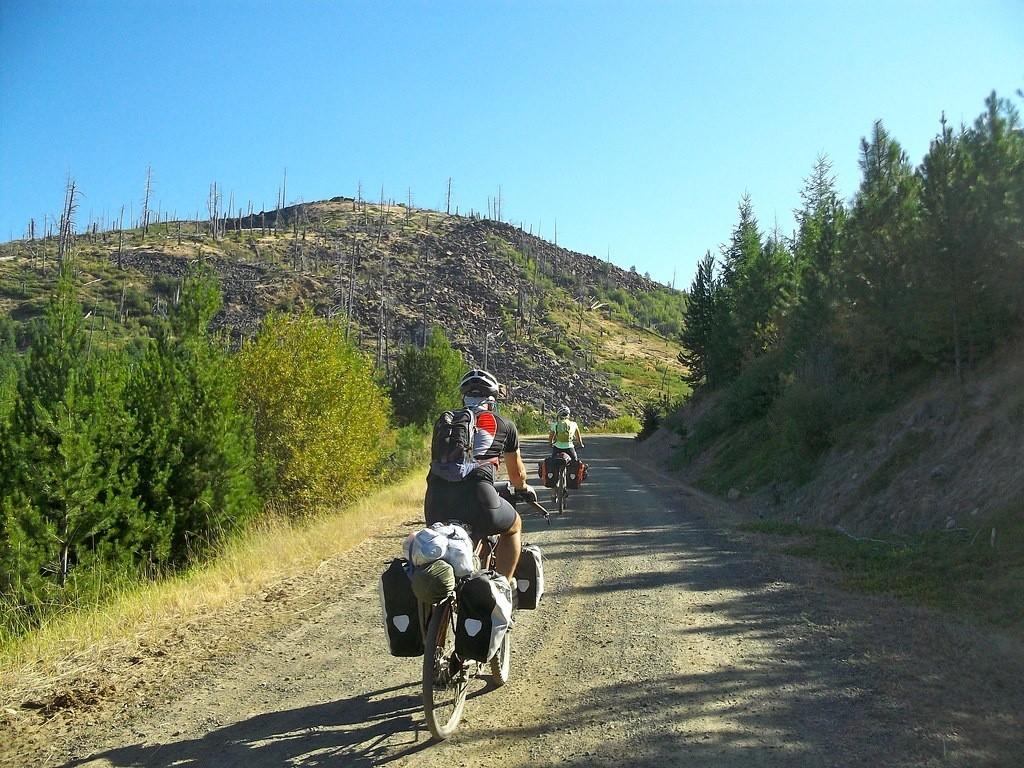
[558,406,570,420]
[459,369,499,399]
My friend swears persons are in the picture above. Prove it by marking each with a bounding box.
[549,406,585,503]
[424,370,537,690]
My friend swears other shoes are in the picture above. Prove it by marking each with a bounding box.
[508,617,515,629]
[563,489,569,497]
[551,493,559,504]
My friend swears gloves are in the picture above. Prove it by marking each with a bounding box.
[578,443,584,448]
[549,443,555,447]
[514,484,537,502]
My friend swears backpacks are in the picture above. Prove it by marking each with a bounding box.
[556,419,572,442]
[431,404,500,481]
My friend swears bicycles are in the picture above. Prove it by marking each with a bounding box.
[422,480,551,740]
[551,445,583,515]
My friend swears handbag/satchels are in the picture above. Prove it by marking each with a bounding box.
[566,458,584,489]
[455,568,512,663]
[379,557,432,656]
[513,540,545,609]
[538,455,558,487]
